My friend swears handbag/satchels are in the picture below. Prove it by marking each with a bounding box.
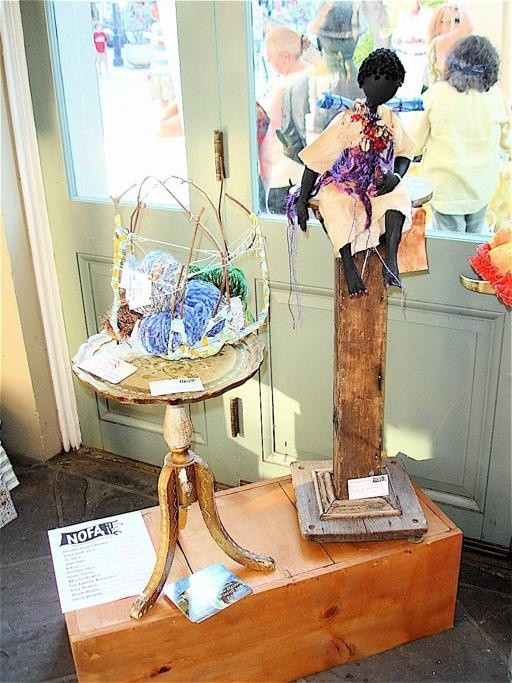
[275,119,304,167]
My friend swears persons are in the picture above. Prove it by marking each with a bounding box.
[296,48,418,298]
[255,2,510,232]
